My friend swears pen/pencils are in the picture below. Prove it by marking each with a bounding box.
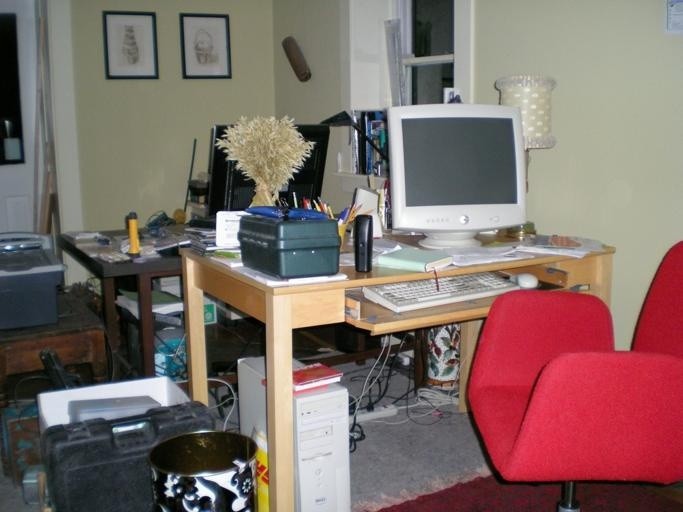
[292,191,363,224]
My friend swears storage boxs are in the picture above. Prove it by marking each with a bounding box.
[36,397,218,512]
[0,241,69,332]
[29,371,192,440]
[235,214,342,280]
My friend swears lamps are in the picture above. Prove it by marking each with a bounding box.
[491,76,560,242]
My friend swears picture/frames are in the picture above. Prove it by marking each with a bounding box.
[175,11,233,81]
[98,8,161,83]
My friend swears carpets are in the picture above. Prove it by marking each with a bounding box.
[0,358,681,512]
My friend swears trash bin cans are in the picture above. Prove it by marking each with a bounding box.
[148,430,258,512]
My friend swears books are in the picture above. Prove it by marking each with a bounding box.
[291,362,343,391]
[516,232,603,259]
[442,87,462,104]
[111,289,184,320]
[379,248,453,273]
[351,184,379,220]
[351,108,388,175]
[65,189,351,298]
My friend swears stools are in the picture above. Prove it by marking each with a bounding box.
[0,296,117,435]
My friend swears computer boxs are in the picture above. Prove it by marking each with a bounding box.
[122,308,188,382]
[238,356,352,512]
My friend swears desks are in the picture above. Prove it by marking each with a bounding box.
[54,222,428,405]
[176,234,614,510]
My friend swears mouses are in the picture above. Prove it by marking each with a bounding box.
[510,273,539,289]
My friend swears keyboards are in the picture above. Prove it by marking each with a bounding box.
[362,272,520,314]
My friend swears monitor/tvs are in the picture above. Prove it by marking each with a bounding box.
[387,104,527,250]
[206,124,329,215]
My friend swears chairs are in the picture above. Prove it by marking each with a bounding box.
[462,239,682,511]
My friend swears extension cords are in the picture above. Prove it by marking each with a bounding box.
[349,404,397,424]
[390,353,410,366]
